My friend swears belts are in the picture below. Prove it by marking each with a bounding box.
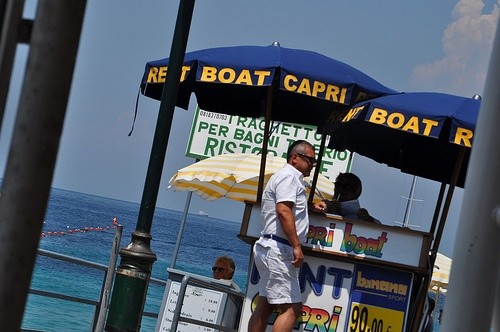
[263,234,292,247]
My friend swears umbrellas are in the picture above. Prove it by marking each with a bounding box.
[129,42,399,203]
[167,155,334,202]
[428,251,452,320]
[327,93,482,332]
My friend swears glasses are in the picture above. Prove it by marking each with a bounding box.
[212,266,229,273]
[292,152,316,164]
[335,181,357,193]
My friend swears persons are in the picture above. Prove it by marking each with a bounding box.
[213,256,235,280]
[324,172,362,217]
[420,296,443,332]
[248,139,327,332]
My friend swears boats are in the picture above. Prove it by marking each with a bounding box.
[197,210,209,217]
